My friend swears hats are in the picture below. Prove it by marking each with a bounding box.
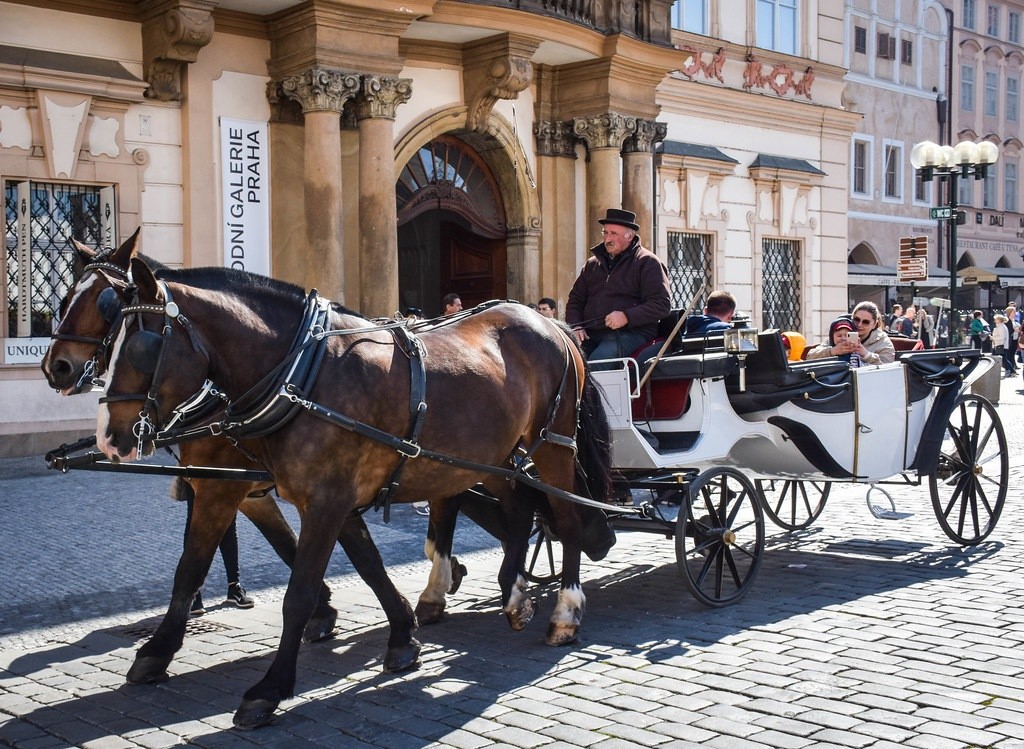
[598,209,640,231]
[835,320,853,332]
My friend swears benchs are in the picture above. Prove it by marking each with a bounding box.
[889,337,924,361]
[747,329,855,413]
[629,307,737,421]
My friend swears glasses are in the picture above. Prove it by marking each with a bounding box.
[853,316,871,325]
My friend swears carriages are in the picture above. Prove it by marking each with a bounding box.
[46,224,1008,731]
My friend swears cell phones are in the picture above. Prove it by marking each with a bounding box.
[846,332,858,345]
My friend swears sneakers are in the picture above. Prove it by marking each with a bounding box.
[190,590,205,614]
[227,583,254,609]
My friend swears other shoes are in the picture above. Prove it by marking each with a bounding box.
[608,496,634,505]
[1008,372,1017,377]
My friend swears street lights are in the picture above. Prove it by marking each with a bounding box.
[911,133,1003,347]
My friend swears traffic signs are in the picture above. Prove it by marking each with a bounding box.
[896,236,926,282]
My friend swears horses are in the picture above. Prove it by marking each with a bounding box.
[38,227,612,732]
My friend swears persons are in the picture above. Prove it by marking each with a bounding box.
[687,290,736,333]
[889,304,934,349]
[807,318,861,368]
[404,293,463,515]
[565,208,671,372]
[538,298,556,318]
[780,331,806,363]
[971,302,1024,377]
[852,301,895,366]
